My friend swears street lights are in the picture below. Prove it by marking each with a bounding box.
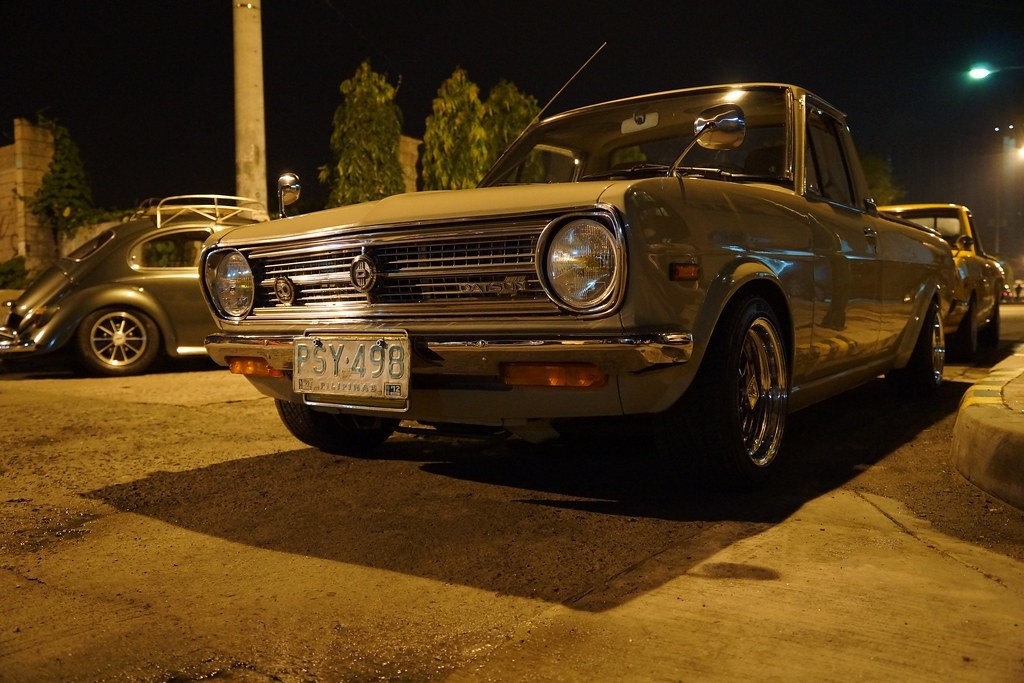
[971,63,1024,80]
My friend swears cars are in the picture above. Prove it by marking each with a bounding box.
[875,203,1009,360]
[173,83,971,509]
[0,193,269,377]
